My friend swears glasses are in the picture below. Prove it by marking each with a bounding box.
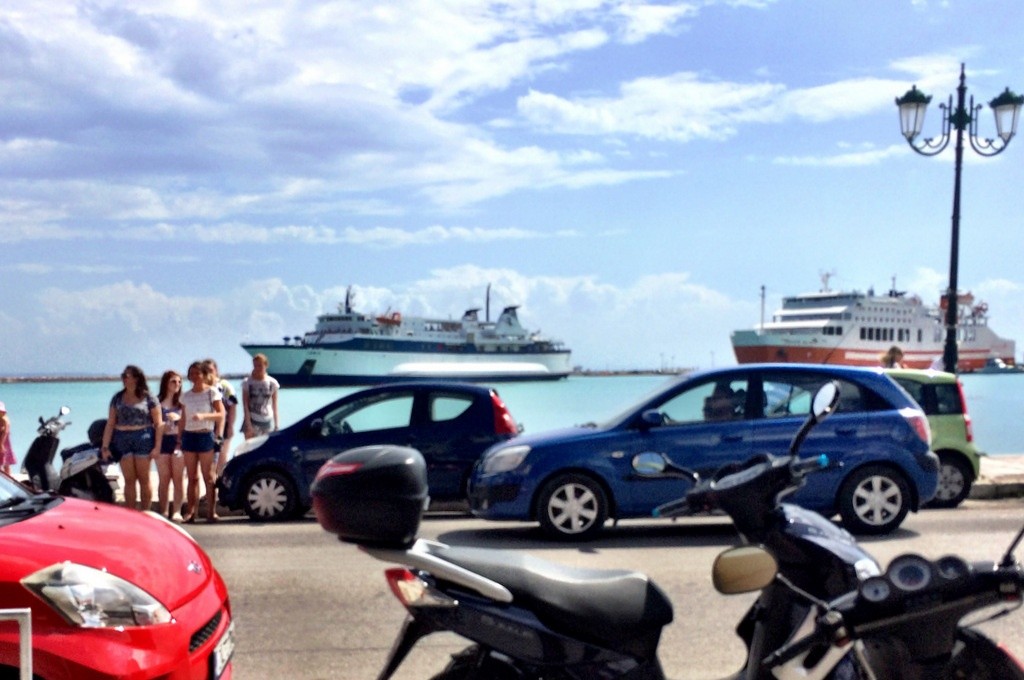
[121,372,134,379]
[170,380,182,384]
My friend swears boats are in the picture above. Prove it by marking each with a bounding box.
[731,275,1024,377]
[240,284,575,387]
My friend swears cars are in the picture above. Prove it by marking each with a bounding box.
[466,362,942,543]
[214,382,525,525]
[773,371,983,508]
[0,470,235,680]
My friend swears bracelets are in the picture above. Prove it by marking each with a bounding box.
[103,447,109,451]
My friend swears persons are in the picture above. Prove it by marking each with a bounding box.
[240,354,280,439]
[203,360,238,474]
[102,366,163,510]
[153,371,185,520]
[882,346,907,369]
[176,362,226,523]
[703,385,735,418]
[0,402,16,474]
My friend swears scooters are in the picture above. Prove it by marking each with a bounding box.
[308,380,1022,680]
[18,405,121,503]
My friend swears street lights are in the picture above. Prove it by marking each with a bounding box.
[893,62,1024,373]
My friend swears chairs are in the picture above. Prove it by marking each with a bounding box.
[732,389,766,420]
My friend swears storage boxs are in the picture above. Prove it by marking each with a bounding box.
[308,444,430,548]
[88,420,108,446]
